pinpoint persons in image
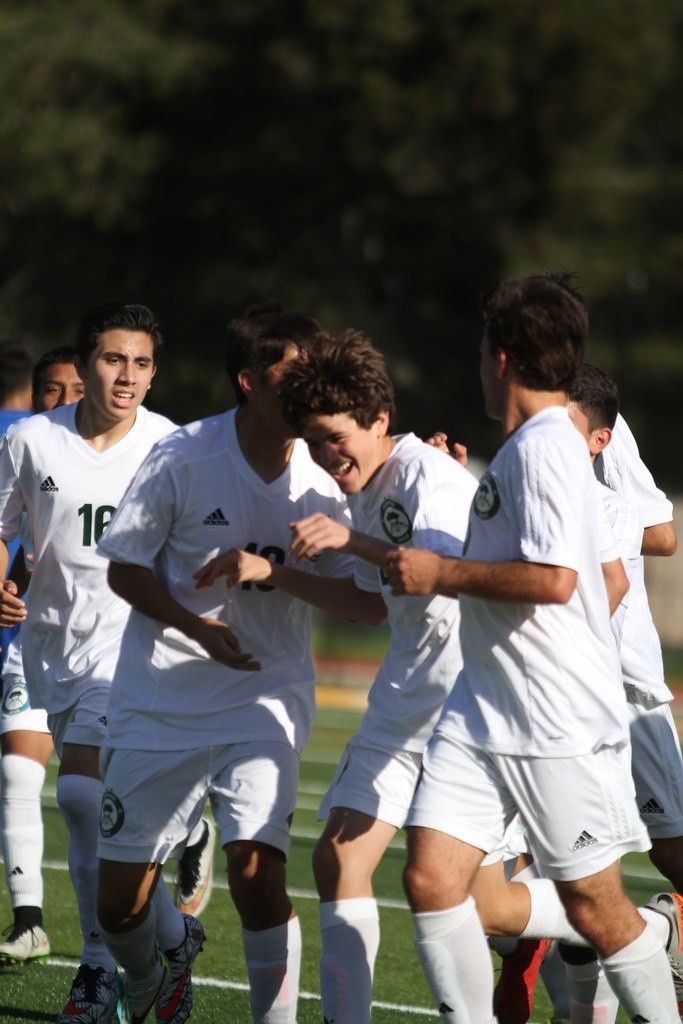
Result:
[0,277,683,1024]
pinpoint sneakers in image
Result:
[153,915,208,1024]
[57,964,122,1024]
[172,818,216,918]
[493,939,553,1024]
[0,923,50,966]
[645,892,683,1021]
[122,950,170,1024]
